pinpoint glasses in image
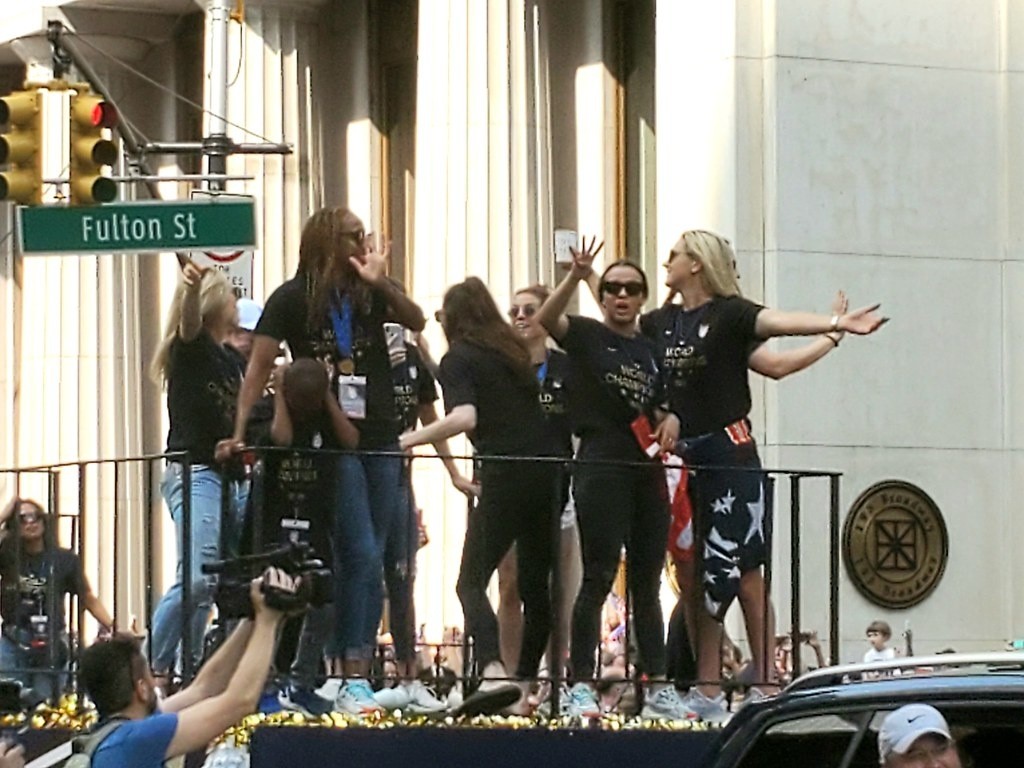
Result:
[435,310,446,322]
[507,307,535,318]
[230,287,241,299]
[668,250,694,263]
[603,281,644,298]
[336,229,365,246]
[19,511,44,524]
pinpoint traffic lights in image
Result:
[0,92,44,207]
[69,95,119,207]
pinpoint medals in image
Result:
[337,358,354,374]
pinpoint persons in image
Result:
[879,702,964,768]
[1,206,890,716]
[862,621,1018,679]
[72,564,298,767]
[0,741,25,768]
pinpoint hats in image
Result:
[236,298,263,331]
[878,704,951,764]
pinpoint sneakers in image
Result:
[254,672,769,727]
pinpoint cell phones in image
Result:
[383,324,404,355]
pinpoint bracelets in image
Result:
[826,334,838,346]
[831,315,838,329]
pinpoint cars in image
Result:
[692,650,1024,768]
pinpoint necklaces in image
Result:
[678,298,709,346]
[666,307,709,378]
